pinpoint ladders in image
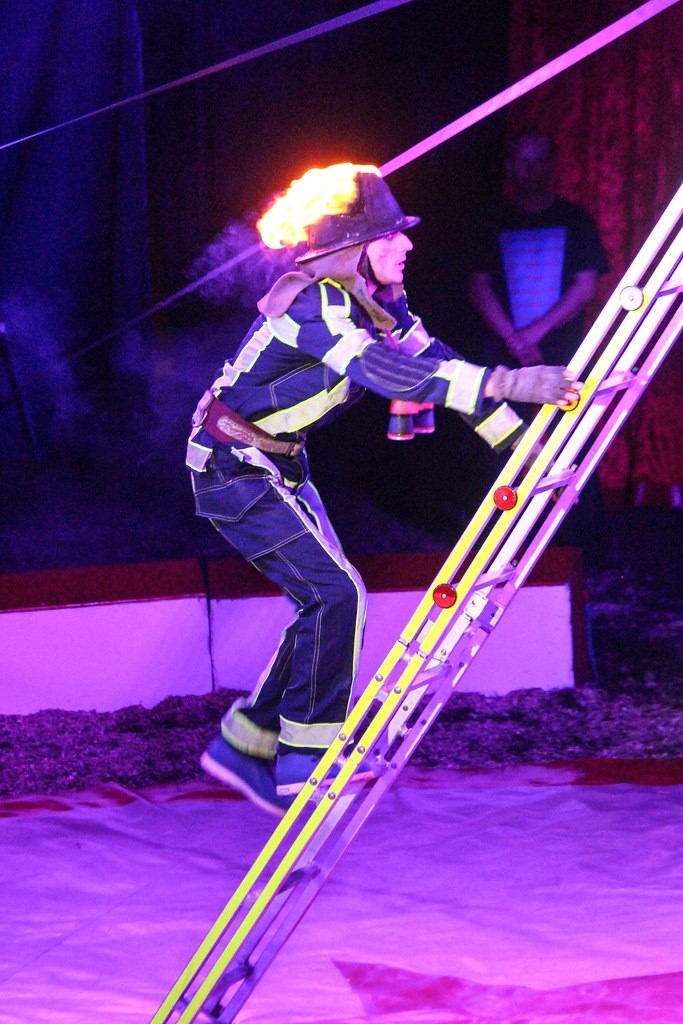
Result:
[147,188,683,1024]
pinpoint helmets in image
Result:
[295,172,421,263]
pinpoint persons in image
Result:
[184,161,594,818]
[460,127,611,557]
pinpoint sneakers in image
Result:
[201,735,295,817]
[275,742,374,797]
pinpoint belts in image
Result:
[216,416,303,457]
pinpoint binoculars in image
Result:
[386,398,435,440]
